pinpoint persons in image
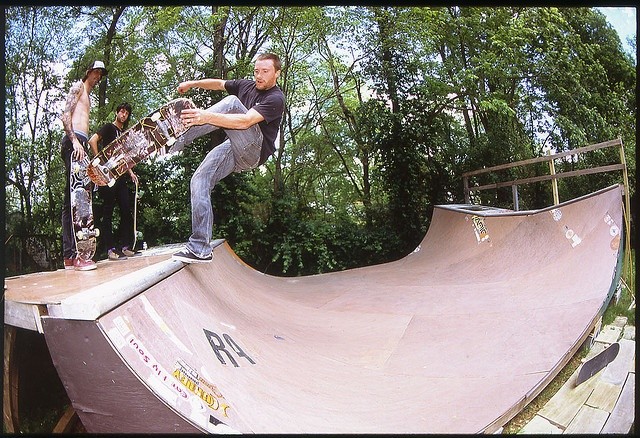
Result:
[156,52,287,264]
[61,60,109,271]
[87,103,143,260]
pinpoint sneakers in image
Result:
[64,257,74,269]
[73,258,97,270]
[122,246,142,256]
[159,145,170,155]
[108,247,127,259]
[172,247,213,263]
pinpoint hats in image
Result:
[88,60,108,75]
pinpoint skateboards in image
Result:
[87,97,199,186]
[70,150,100,262]
[575,343,620,387]
[132,179,144,250]
